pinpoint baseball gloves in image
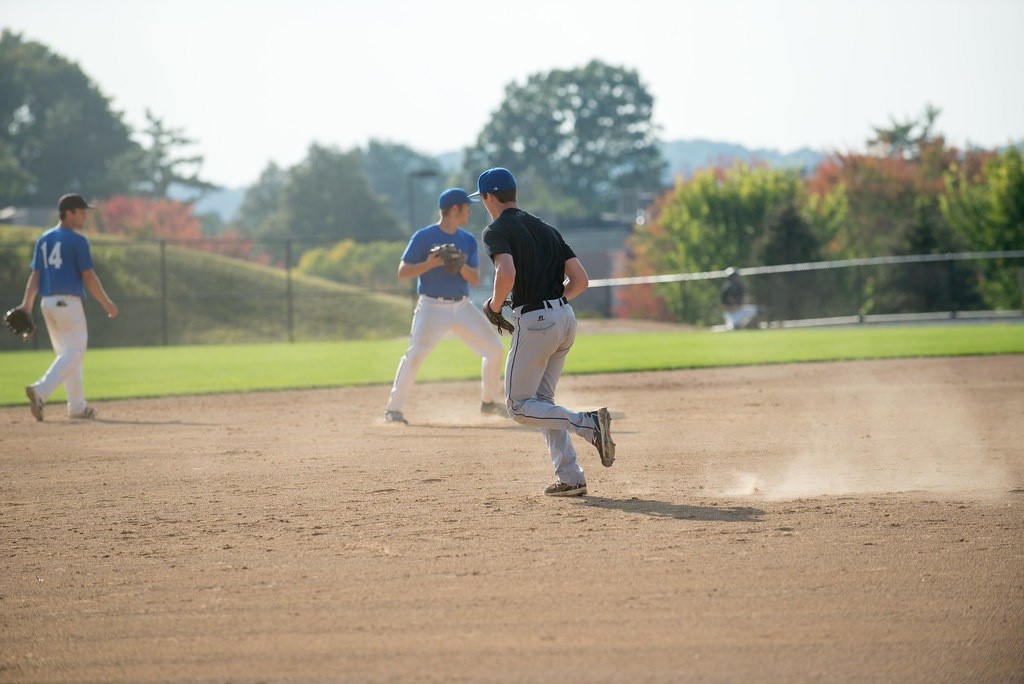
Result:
[3,307,37,341]
[430,244,465,274]
[482,296,516,335]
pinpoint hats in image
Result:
[439,188,480,207]
[467,168,515,199]
[58,194,95,210]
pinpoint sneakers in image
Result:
[27,386,43,422]
[69,405,98,420]
[585,407,615,467]
[544,482,587,497]
[385,411,408,426]
[480,402,511,419]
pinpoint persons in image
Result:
[467,167,617,495]
[387,189,508,425]
[4,193,119,421]
[713,268,757,333]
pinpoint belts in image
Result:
[520,297,568,315]
[425,294,462,302]
